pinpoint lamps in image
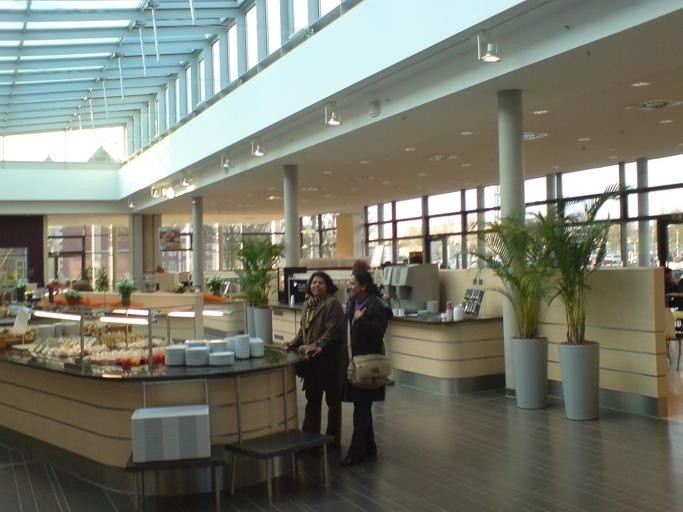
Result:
[220,152,231,169]
[127,197,135,208]
[474,31,500,62]
[250,138,264,157]
[323,104,342,128]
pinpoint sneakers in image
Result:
[342,447,376,466]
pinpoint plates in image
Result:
[408,300,439,318]
[164,335,264,368]
[12,344,29,351]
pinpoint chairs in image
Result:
[225,365,335,508]
[665,307,682,372]
[125,378,226,511]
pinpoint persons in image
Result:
[282,270,348,451]
[663,266,679,308]
[346,258,395,386]
[338,269,391,468]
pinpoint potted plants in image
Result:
[206,276,224,297]
[116,272,136,306]
[528,183,632,421]
[235,237,285,343]
[451,217,553,410]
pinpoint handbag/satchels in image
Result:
[348,354,391,389]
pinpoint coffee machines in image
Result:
[382,263,441,314]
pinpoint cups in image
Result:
[440,303,464,322]
[408,251,424,264]
[391,308,407,318]
[289,295,295,307]
